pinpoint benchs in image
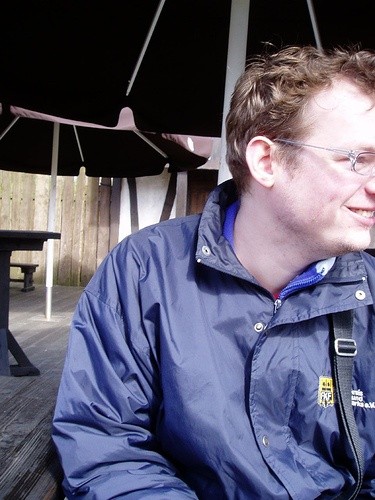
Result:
[9,263,41,293]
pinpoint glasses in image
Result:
[271,137,375,175]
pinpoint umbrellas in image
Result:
[0,0,375,184]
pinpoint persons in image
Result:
[51,45,375,500]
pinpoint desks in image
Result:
[0,229,63,378]
